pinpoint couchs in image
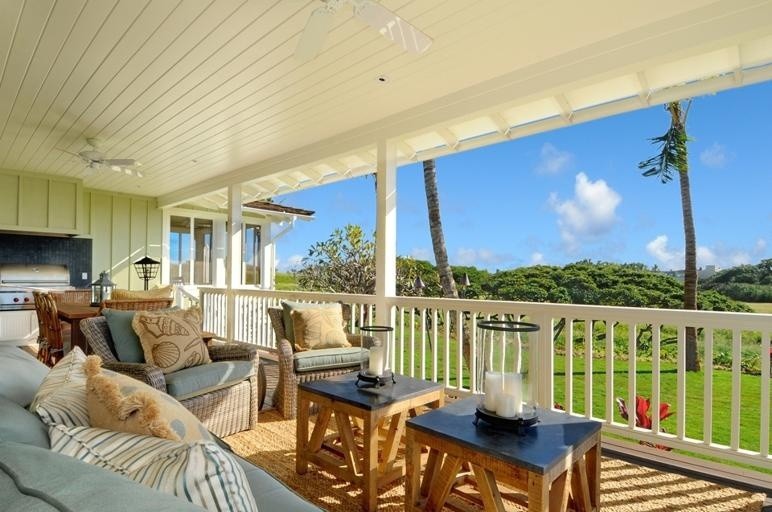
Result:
[0,344,325,512]
[79,309,261,440]
[267,302,381,419]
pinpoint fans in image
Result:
[60,138,146,181]
[292,1,434,60]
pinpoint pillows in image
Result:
[287,307,353,351]
[45,421,257,512]
[102,311,146,363]
[29,344,112,429]
[280,299,341,350]
[133,310,213,374]
[83,355,216,441]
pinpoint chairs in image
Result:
[30,288,45,362]
[103,297,174,312]
[42,292,64,367]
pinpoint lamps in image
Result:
[133,257,161,291]
